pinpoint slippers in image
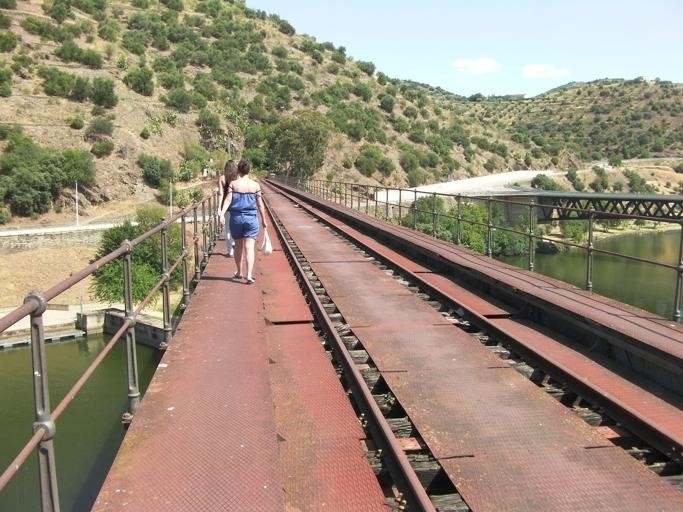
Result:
[234,271,255,284]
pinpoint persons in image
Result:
[219,160,239,259]
[219,159,267,284]
